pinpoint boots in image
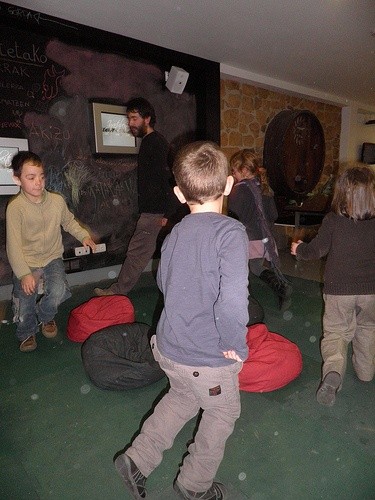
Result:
[258,267,294,312]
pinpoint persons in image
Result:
[226,148,295,324]
[113,140,250,500]
[4,150,97,354]
[290,164,374,409]
[93,96,179,299]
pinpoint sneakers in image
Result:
[114,453,147,500]
[19,334,36,353]
[315,371,341,408]
[41,318,57,338]
[171,480,226,500]
[95,287,123,299]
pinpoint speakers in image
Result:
[164,65,189,94]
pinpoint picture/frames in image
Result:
[87,96,143,160]
[0,137,30,196]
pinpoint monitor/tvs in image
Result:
[91,101,141,157]
